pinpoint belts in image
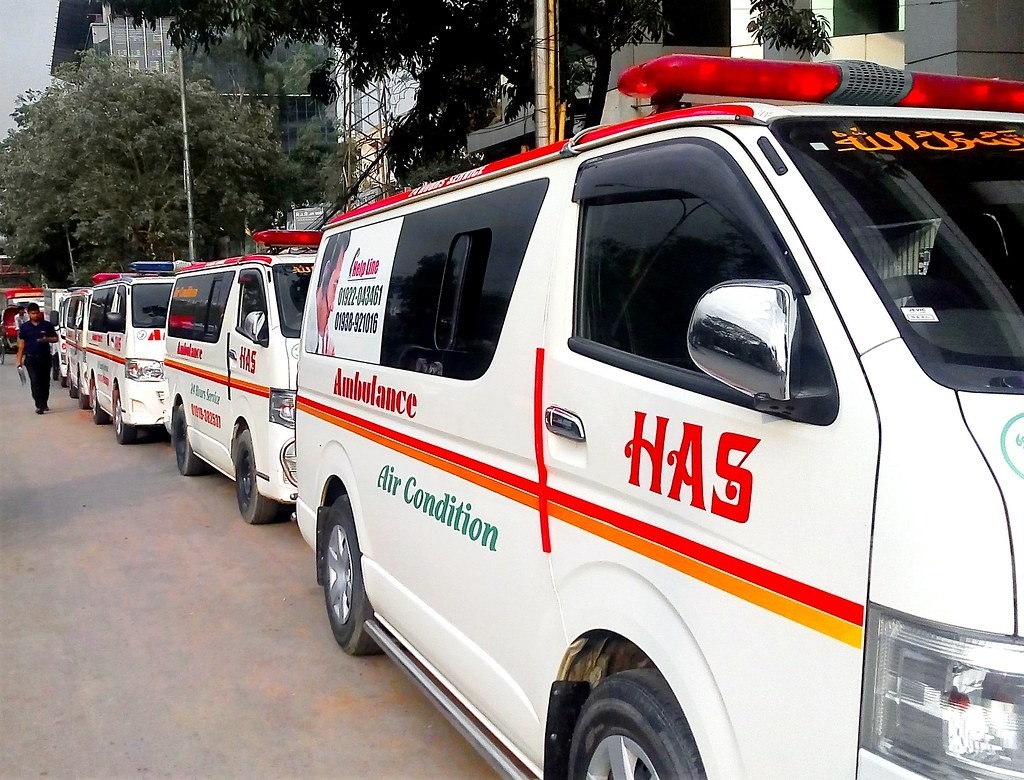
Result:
[26,354,48,357]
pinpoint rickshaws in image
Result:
[0,306,31,364]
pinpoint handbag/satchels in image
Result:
[17,366,26,383]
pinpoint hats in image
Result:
[18,306,25,311]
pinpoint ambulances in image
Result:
[0,260,206,446]
[162,230,327,526]
[293,53,1024,780]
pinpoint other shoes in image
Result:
[35,408,43,414]
[43,407,48,411]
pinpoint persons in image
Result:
[14,306,30,331]
[17,302,59,414]
[306,230,352,355]
